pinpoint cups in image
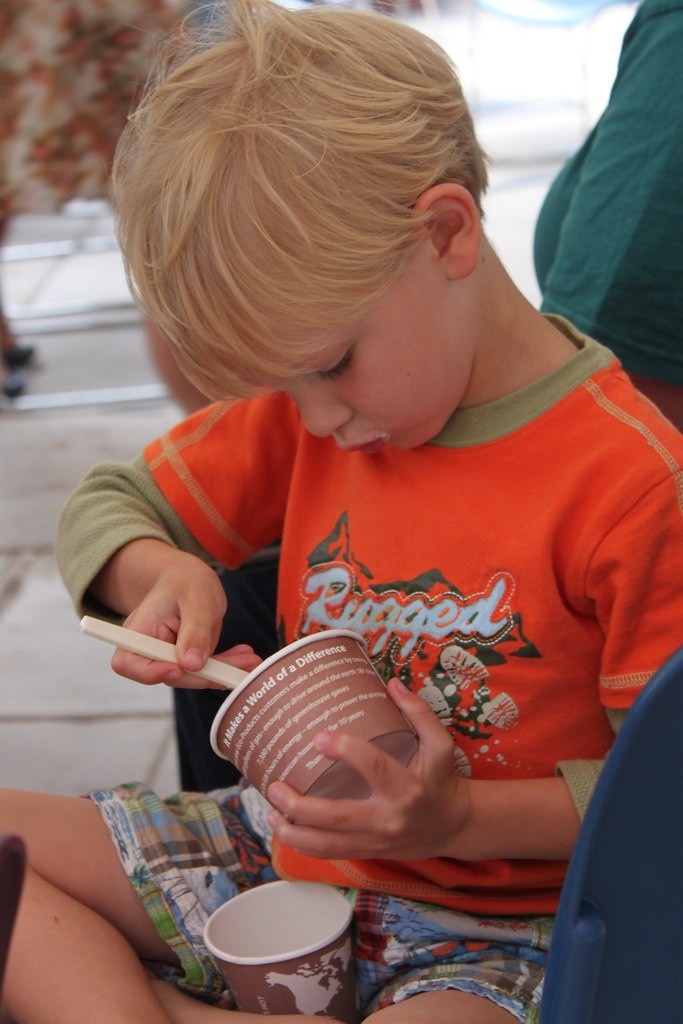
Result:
[202,880,364,1024]
[209,628,419,825]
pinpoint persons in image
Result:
[168,0,682,791]
[0,0,683,1024]
